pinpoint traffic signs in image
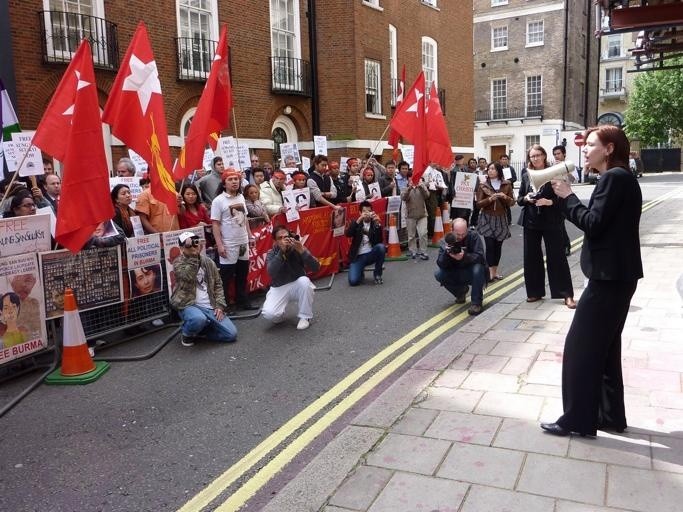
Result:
[574,135,586,147]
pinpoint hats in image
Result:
[220,168,241,184]
[454,154,464,160]
[176,231,199,246]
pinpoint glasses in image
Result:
[15,202,35,207]
[529,153,543,158]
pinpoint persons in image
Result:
[35,174,62,211]
[261,225,320,330]
[200,155,225,211]
[107,160,145,235]
[107,185,157,236]
[177,184,210,227]
[433,217,485,315]
[238,183,272,226]
[521,150,533,171]
[337,158,359,201]
[365,153,386,182]
[132,267,156,297]
[377,161,400,196]
[496,155,517,223]
[402,172,429,261]
[539,125,643,439]
[551,148,581,257]
[5,193,35,218]
[38,154,52,180]
[289,171,309,190]
[395,160,409,196]
[327,160,346,202]
[423,162,443,239]
[171,231,239,348]
[283,156,299,172]
[443,155,486,226]
[474,163,513,283]
[135,183,182,232]
[309,156,343,212]
[333,208,345,230]
[343,201,387,286]
[358,167,375,200]
[0,180,26,206]
[260,170,292,214]
[0,272,40,350]
[243,156,266,188]
[210,168,259,315]
[519,146,579,311]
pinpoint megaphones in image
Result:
[525,161,576,194]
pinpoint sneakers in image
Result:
[469,225,475,230]
[271,318,281,324]
[87,346,95,359]
[468,302,483,315]
[361,271,365,280]
[411,252,416,259]
[297,319,310,329]
[95,339,106,346]
[419,253,429,260]
[374,275,384,284]
[158,319,164,325]
[454,284,468,303]
[181,333,194,347]
[153,320,160,326]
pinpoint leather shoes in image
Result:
[564,297,575,309]
[237,303,260,310]
[526,296,541,301]
[135,326,145,332]
[541,422,592,438]
[487,273,495,282]
[123,327,136,336]
[596,423,625,433]
[495,272,503,279]
[225,306,235,316]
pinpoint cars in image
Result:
[596,154,644,182]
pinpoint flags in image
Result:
[32,39,114,255]
[170,22,231,182]
[102,19,179,215]
[391,69,426,185]
[413,80,452,185]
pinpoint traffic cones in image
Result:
[427,207,445,248]
[382,212,409,262]
[442,202,452,235]
[43,288,113,385]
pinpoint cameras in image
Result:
[188,239,205,246]
[445,232,462,254]
[366,211,374,218]
[288,231,300,240]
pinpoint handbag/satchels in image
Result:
[484,178,512,225]
